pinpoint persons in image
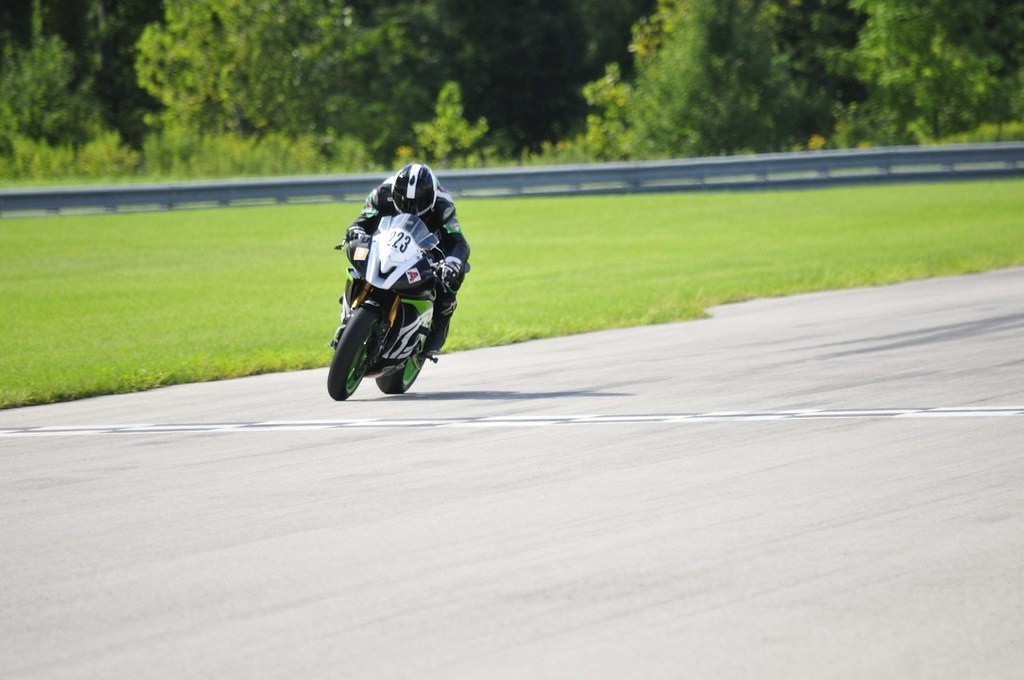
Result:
[345,163,471,354]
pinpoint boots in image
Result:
[424,302,456,352]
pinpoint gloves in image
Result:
[344,225,368,245]
[434,256,463,285]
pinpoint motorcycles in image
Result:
[327,213,470,403]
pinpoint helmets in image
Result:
[389,163,438,216]
